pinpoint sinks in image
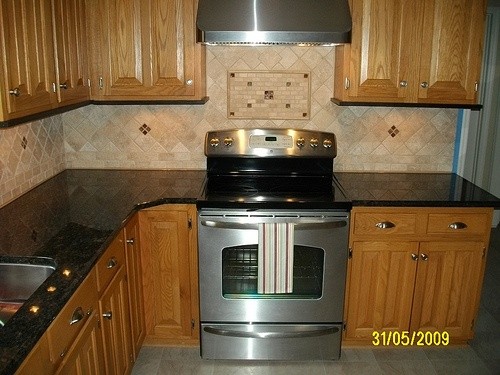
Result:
[0,255,57,329]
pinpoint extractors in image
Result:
[195,0,353,46]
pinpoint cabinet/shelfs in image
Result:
[345,208,489,348]
[86,2,196,96]
[334,0,488,103]
[140,208,190,337]
[0,0,86,114]
[19,215,144,372]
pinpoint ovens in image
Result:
[197,214,351,360]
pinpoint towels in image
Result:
[257,223,294,294]
